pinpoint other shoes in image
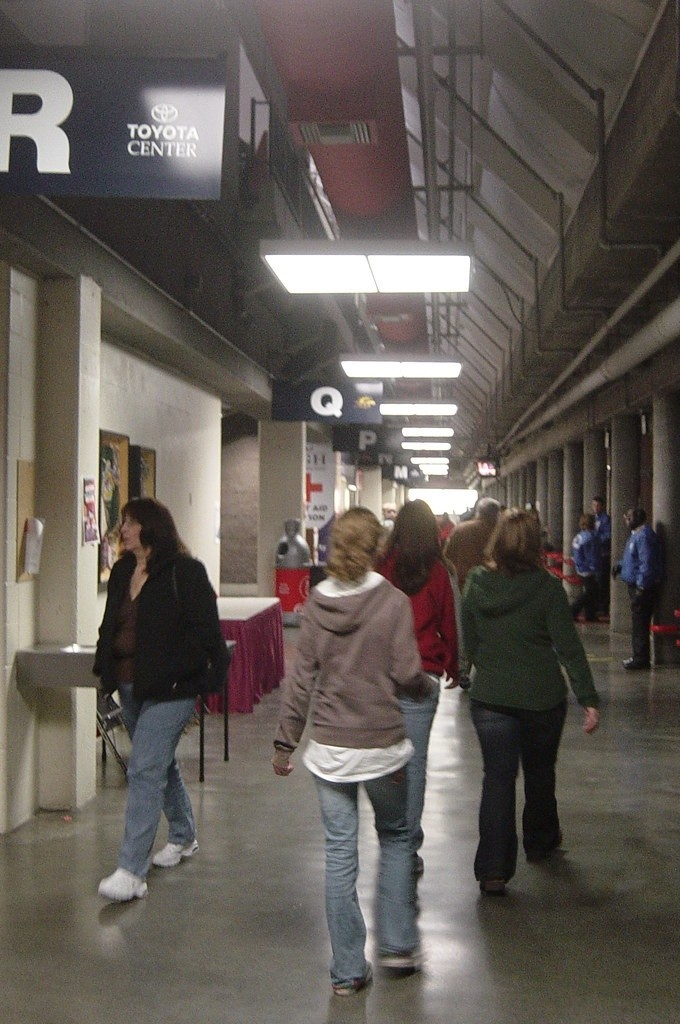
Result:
[527,830,562,858]
[479,880,504,892]
[623,657,650,669]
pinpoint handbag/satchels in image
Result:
[172,556,230,693]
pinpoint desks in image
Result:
[196,596,286,715]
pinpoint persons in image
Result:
[375,495,459,873]
[274,519,311,568]
[610,506,662,672]
[455,507,603,895]
[273,506,434,995]
[435,498,508,594]
[92,499,229,902]
[568,496,610,623]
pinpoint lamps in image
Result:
[337,352,463,379]
[259,237,471,294]
[380,398,459,417]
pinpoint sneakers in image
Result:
[98,867,148,901]
[152,838,199,866]
[376,949,422,968]
[335,962,372,996]
